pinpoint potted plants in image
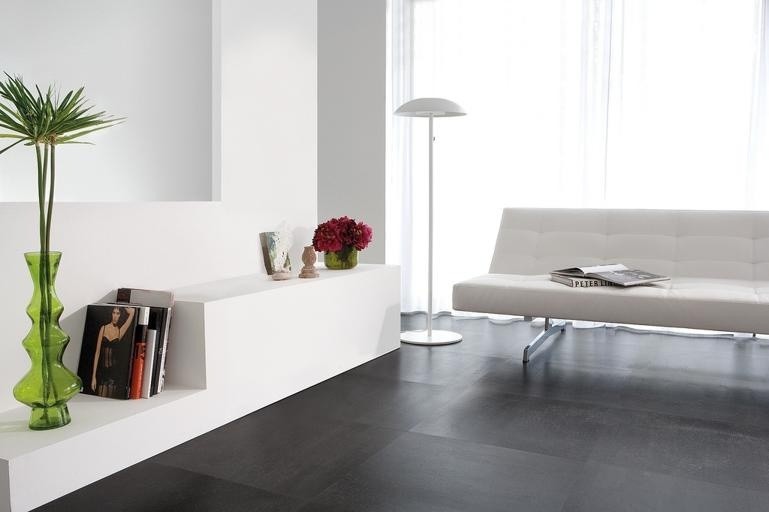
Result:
[0,72,128,434]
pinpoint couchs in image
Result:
[453,207,769,367]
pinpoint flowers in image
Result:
[312,213,374,270]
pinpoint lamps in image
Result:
[392,96,466,346]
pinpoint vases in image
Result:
[322,245,359,269]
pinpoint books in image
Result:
[72,288,179,403]
[259,230,294,275]
[550,262,673,287]
[550,275,617,289]
